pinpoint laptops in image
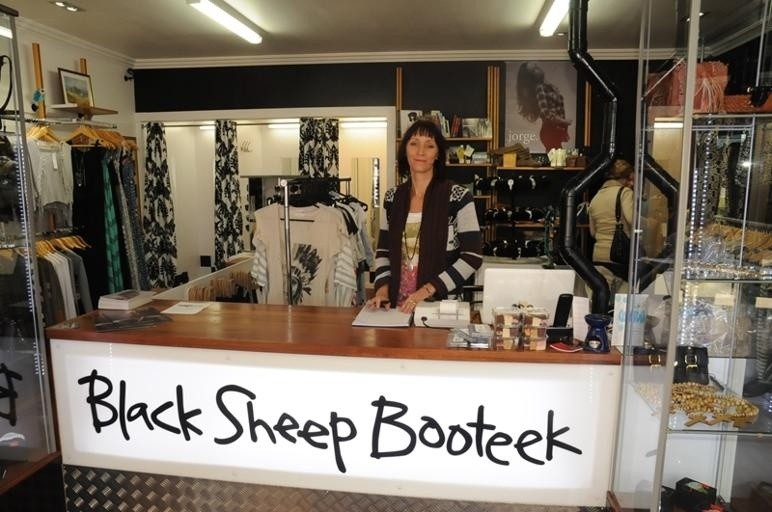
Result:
[479,268,577,325]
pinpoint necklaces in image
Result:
[402,220,421,271]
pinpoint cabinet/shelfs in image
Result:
[395,133,586,261]
[603,109,772,512]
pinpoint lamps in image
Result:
[187,0,265,45]
[538,0,570,38]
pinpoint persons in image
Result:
[588,160,634,282]
[366,121,484,314]
[517,62,573,152]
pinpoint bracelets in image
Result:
[423,284,432,298]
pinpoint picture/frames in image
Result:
[57,67,95,107]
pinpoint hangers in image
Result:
[257,174,368,217]
[0,229,94,265]
[188,269,256,302]
[20,120,71,149]
[63,120,140,151]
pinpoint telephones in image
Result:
[414,300,471,328]
